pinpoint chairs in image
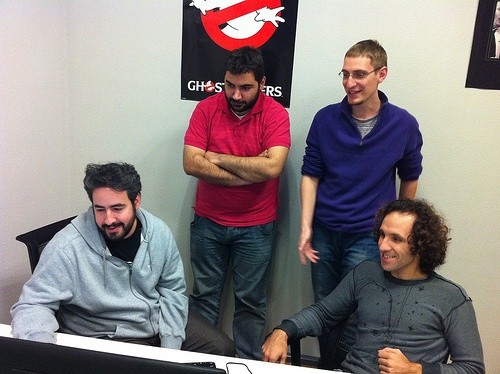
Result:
[16,216,77,275]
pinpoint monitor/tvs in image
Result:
[0,337,227,374]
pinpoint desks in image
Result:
[0,324,350,374]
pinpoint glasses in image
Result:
[338,65,385,80]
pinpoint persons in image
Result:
[183,46,292,362]
[261,197,486,374]
[10,161,237,357]
[298,39,423,369]
